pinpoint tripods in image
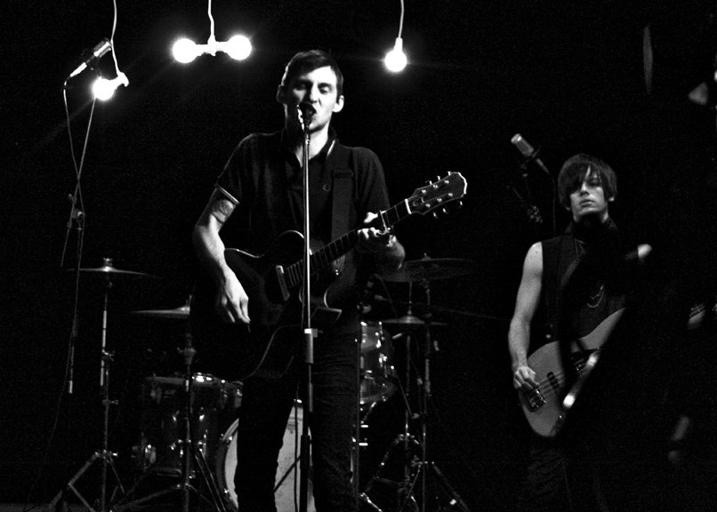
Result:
[108,324,227,512]
[360,284,465,512]
[48,279,126,512]
[399,292,471,512]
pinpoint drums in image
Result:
[141,372,235,477]
[216,399,361,507]
[359,321,397,405]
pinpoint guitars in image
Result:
[188,171,467,387]
[517,303,716,438]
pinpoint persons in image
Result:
[190,50,406,511]
[506,154,686,512]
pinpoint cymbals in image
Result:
[379,258,478,282]
[127,306,190,321]
[377,316,447,326]
[73,267,153,277]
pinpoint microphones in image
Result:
[510,133,549,173]
[67,38,113,80]
[296,101,316,128]
